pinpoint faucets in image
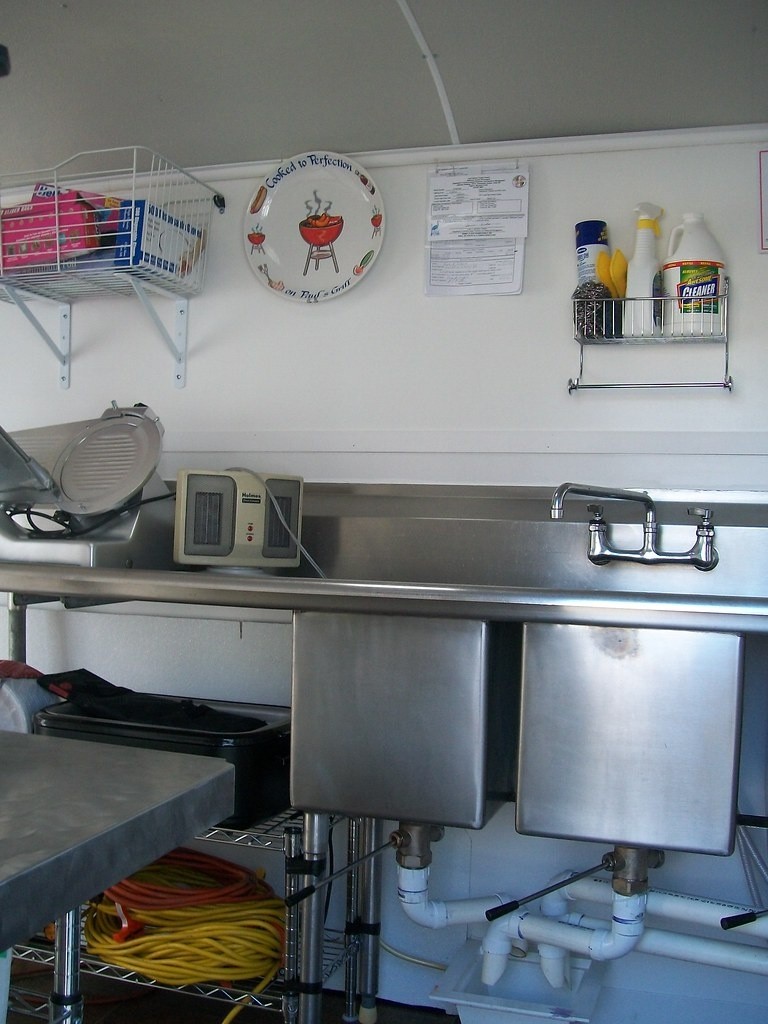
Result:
[551,483,658,553]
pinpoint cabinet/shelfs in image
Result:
[9,810,360,1024]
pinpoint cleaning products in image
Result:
[662,210,729,338]
[620,202,665,338]
[576,218,613,340]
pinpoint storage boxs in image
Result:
[29,696,296,828]
[0,141,220,303]
[567,276,729,342]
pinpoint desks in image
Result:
[0,730,242,1024]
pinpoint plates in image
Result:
[243,150,385,303]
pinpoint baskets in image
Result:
[0,146,223,306]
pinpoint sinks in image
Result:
[289,573,511,831]
[516,585,745,857]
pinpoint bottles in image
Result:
[624,202,665,336]
[662,212,725,335]
